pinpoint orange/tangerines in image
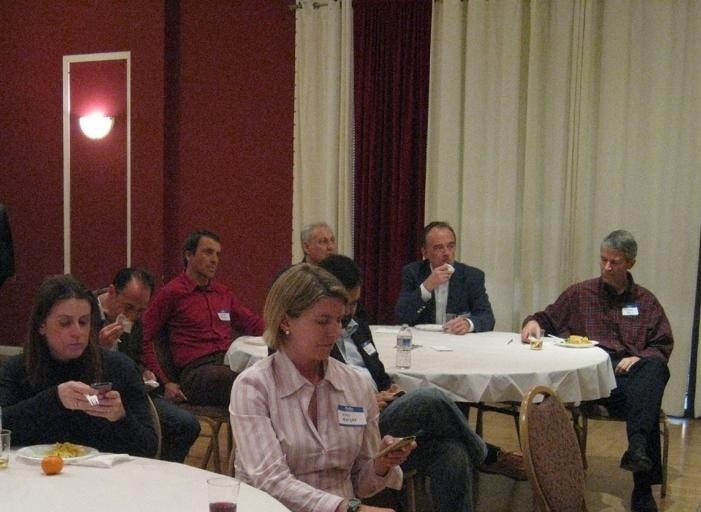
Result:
[40,456,62,476]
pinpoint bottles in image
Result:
[396,324,413,370]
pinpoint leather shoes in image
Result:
[618,450,654,472]
[480,441,529,481]
[630,489,658,512]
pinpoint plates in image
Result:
[415,324,444,331]
[246,337,265,345]
[557,340,599,348]
[16,444,98,465]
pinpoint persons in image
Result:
[521,229,674,512]
[393,222,495,418]
[322,257,527,512]
[87,269,201,463]
[143,228,266,408]
[228,263,417,512]
[0,275,159,457]
[278,223,337,266]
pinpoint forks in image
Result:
[83,393,99,406]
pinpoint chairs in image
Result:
[519,384,589,512]
[573,401,669,498]
[154,334,230,473]
[147,395,162,460]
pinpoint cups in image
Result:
[207,477,240,512]
[0,430,10,468]
[91,382,113,395]
[443,313,455,328]
[530,330,545,350]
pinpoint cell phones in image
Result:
[89,380,109,394]
[367,433,419,463]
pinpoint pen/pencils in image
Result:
[507,339,513,345]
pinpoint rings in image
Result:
[73,403,79,409]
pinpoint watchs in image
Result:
[347,497,361,512]
